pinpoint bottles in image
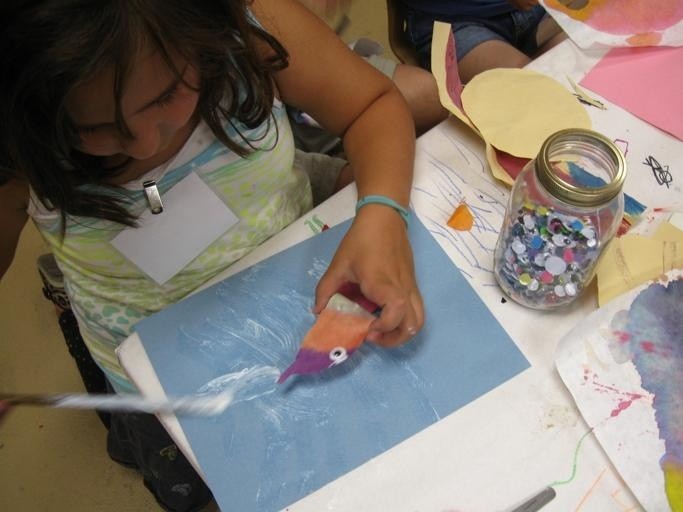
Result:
[491,126,626,311]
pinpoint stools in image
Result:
[34,253,113,436]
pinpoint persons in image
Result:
[284,35,451,208]
[389,0,566,84]
[1,0,426,512]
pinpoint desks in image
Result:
[113,26,683,512]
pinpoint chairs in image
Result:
[383,0,425,71]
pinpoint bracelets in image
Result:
[355,194,412,231]
